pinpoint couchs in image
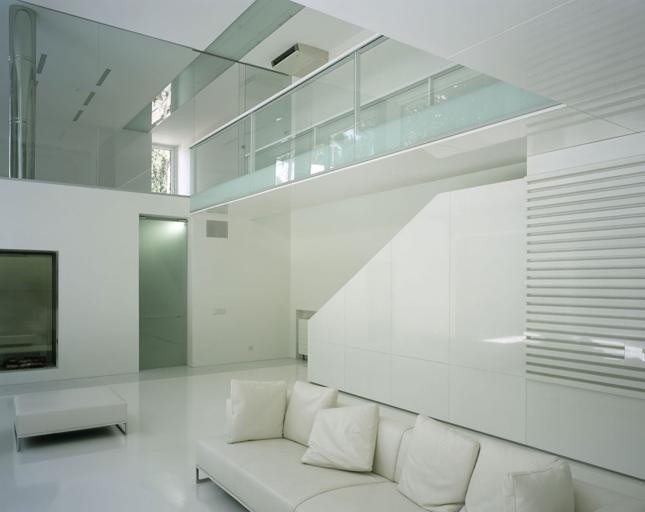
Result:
[196,378,645,512]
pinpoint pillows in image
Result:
[503,457,576,512]
[224,379,378,472]
[396,411,480,512]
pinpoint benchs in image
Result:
[11,385,129,451]
